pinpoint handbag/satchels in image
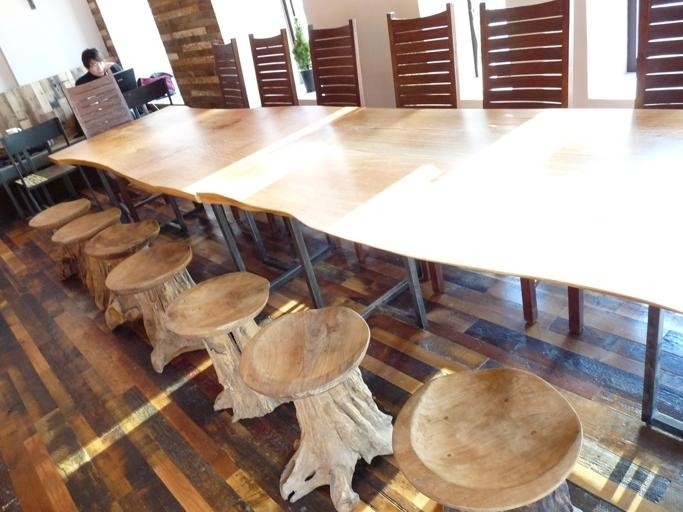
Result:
[137,72,175,99]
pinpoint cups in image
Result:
[4,127,21,137]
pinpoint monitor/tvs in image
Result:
[113,68,137,94]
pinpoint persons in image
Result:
[74,48,145,120]
